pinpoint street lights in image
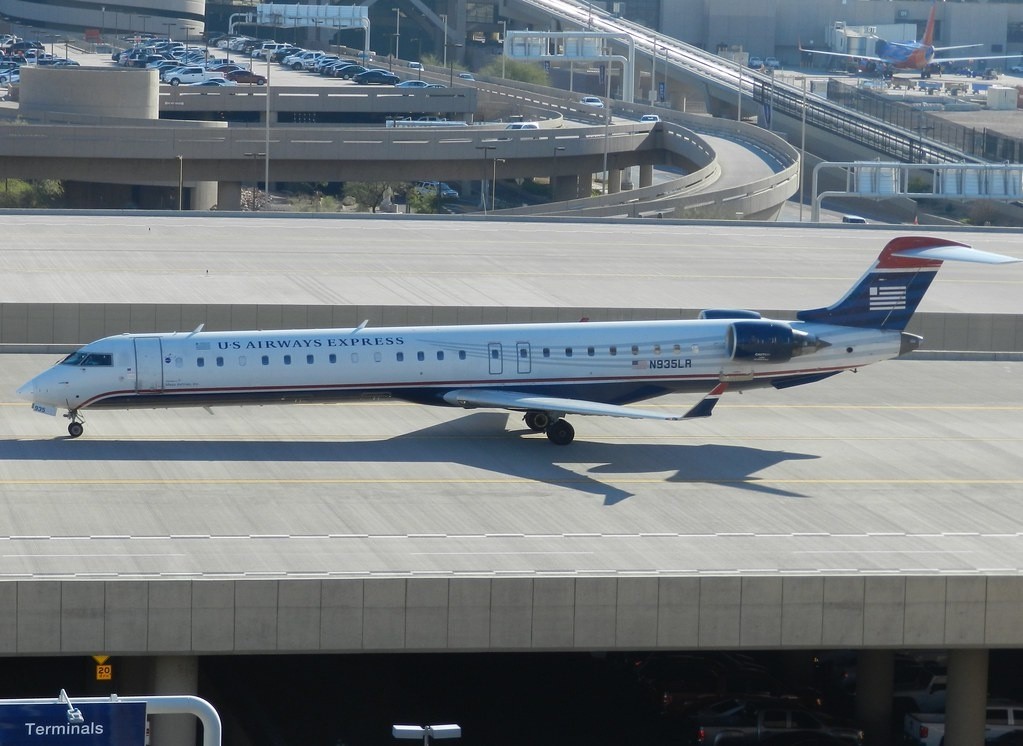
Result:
[176,156,184,211]
[498,21,507,80]
[492,157,505,212]
[440,13,448,68]
[392,7,400,59]
[245,152,266,211]
[731,45,743,122]
[553,146,566,202]
[659,47,668,103]
[794,76,807,222]
[476,145,497,204]
[648,34,657,108]
[600,45,613,194]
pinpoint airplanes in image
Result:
[797,0,1023,80]
[13,226,1023,447]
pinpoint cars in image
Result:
[496,122,540,142]
[408,62,424,73]
[1012,63,1023,73]
[0,16,469,96]
[358,50,373,62]
[981,67,998,81]
[458,72,475,82]
[639,114,662,134]
[579,97,605,110]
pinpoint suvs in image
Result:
[415,179,459,204]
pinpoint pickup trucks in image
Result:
[765,56,780,69]
[749,57,764,68]
